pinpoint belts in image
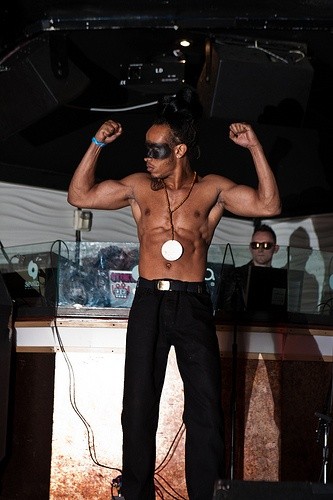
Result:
[139,280,200,293]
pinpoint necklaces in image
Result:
[161,171,197,262]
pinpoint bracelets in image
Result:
[92,137,106,147]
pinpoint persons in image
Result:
[243,224,279,268]
[67,115,282,500]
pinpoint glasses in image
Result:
[250,242,273,250]
